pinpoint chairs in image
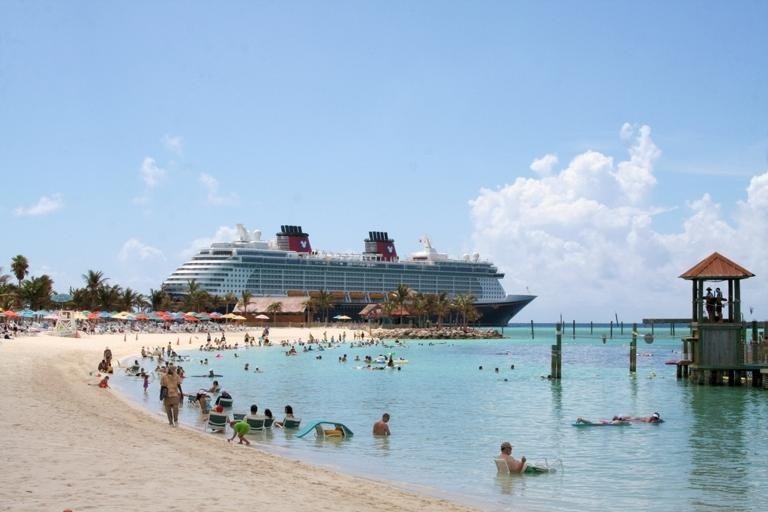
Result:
[206,396,347,441]
[494,459,510,475]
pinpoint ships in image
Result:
[162,224,538,326]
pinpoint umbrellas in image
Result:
[254,313,271,328]
[333,314,352,321]
[0,308,247,321]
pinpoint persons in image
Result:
[3,320,33,337]
[705,287,716,322]
[715,287,724,324]
[196,391,210,414]
[274,405,295,427]
[499,440,557,475]
[477,363,552,381]
[624,412,660,423]
[159,367,185,426]
[762,335,768,363]
[211,405,230,431]
[576,415,625,424]
[372,413,391,436]
[263,408,274,422]
[216,390,233,404]
[122,321,454,392]
[242,404,262,422]
[89,321,95,336]
[98,347,114,388]
[226,419,251,445]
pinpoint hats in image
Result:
[501,441,516,450]
